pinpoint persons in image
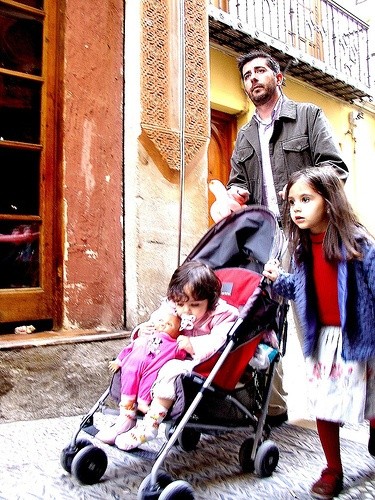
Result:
[107,301,191,433]
[228,49,348,428]
[94,262,242,454]
[262,166,375,496]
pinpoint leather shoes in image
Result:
[253,410,288,433]
[368,433,375,457]
[310,466,344,500]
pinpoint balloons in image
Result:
[209,180,244,222]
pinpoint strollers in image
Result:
[60,205,288,500]
